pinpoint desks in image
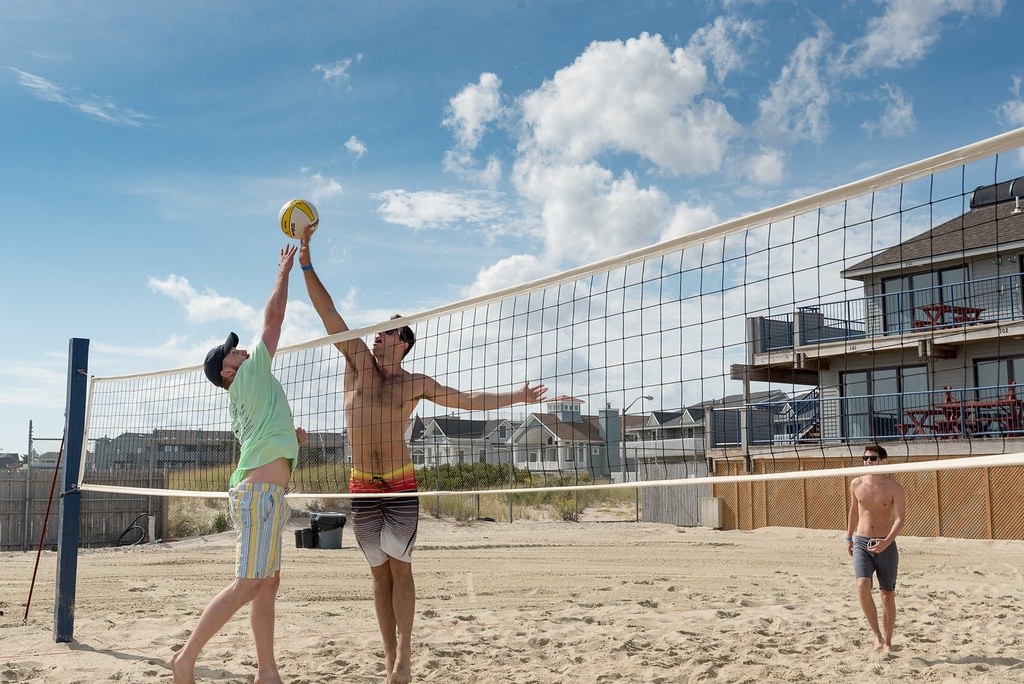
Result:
[906,410,972,441]
[923,305,986,331]
[935,399,1022,439]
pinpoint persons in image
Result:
[846,445,906,654]
[298,222,549,684]
[168,243,302,684]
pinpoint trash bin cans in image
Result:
[294,528,316,548]
[310,512,346,549]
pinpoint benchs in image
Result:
[912,320,946,333]
[953,316,998,327]
[894,417,1023,439]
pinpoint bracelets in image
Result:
[847,537,852,540]
[300,264,313,271]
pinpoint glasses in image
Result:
[861,456,878,461]
[386,328,399,335]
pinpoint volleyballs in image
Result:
[278,198,320,240]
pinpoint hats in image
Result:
[203,332,239,387]
